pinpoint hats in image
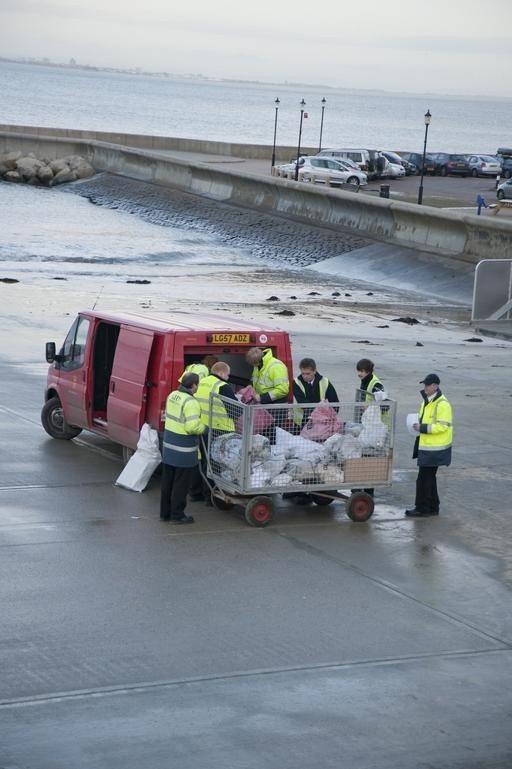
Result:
[357,359,374,370]
[420,374,440,384]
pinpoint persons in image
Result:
[193,361,245,507]
[351,358,390,499]
[405,374,453,517]
[176,354,218,502]
[159,373,206,525]
[281,357,340,499]
[246,347,289,444]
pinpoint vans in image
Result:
[42,309,297,451]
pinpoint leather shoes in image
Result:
[406,509,431,517]
[431,509,438,516]
[191,495,206,501]
[160,516,170,521]
[169,516,192,524]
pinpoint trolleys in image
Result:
[208,394,397,528]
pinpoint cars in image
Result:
[291,135,511,199]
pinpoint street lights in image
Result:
[417,106,432,202]
[318,96,328,153]
[295,98,306,180]
[269,92,280,167]
